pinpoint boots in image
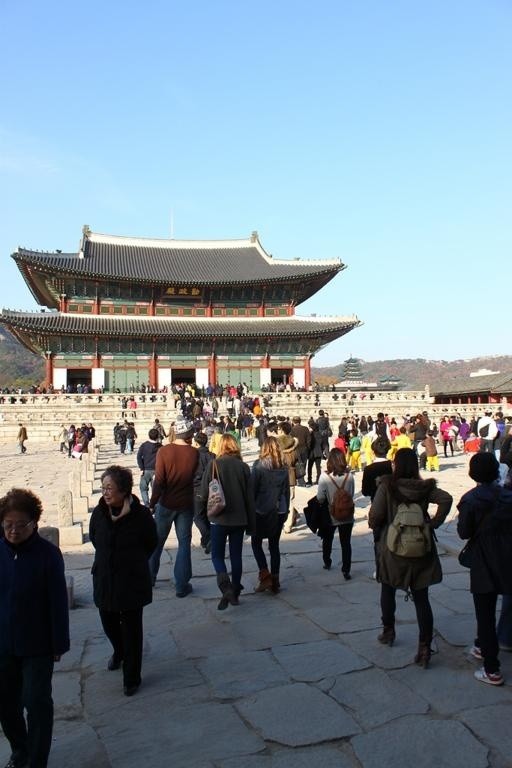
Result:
[217,569,282,610]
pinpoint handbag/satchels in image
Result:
[458,544,485,568]
[207,460,227,518]
[479,424,490,437]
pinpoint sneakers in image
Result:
[468,646,487,666]
[323,563,352,581]
[475,666,504,687]
[497,638,512,652]
[120,449,136,455]
[176,583,195,598]
[19,448,26,455]
[420,453,454,472]
[59,446,82,461]
[307,481,319,486]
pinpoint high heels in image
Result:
[412,642,431,670]
[377,628,397,648]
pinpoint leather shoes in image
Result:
[106,651,122,671]
[123,677,141,697]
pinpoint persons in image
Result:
[56,379,511,612]
[0,488,72,768]
[89,466,159,698]
[16,423,29,454]
[366,446,454,671]
[455,450,512,688]
[495,467,511,657]
[1,383,56,405]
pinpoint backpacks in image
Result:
[383,500,431,558]
[322,469,356,523]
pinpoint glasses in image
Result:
[0,520,35,530]
[100,485,127,495]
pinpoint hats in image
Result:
[173,418,195,439]
[466,451,502,483]
